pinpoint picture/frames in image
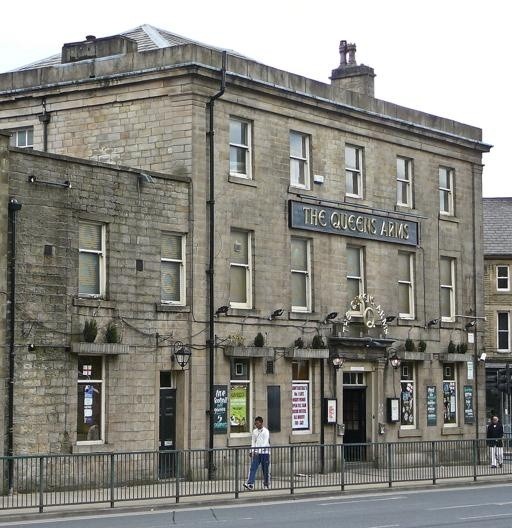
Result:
[322,397,338,425]
[386,397,401,423]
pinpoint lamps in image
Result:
[327,351,346,398]
[387,351,404,397]
[212,306,479,332]
[171,340,192,372]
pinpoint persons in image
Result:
[243,415,270,490]
[486,416,505,468]
